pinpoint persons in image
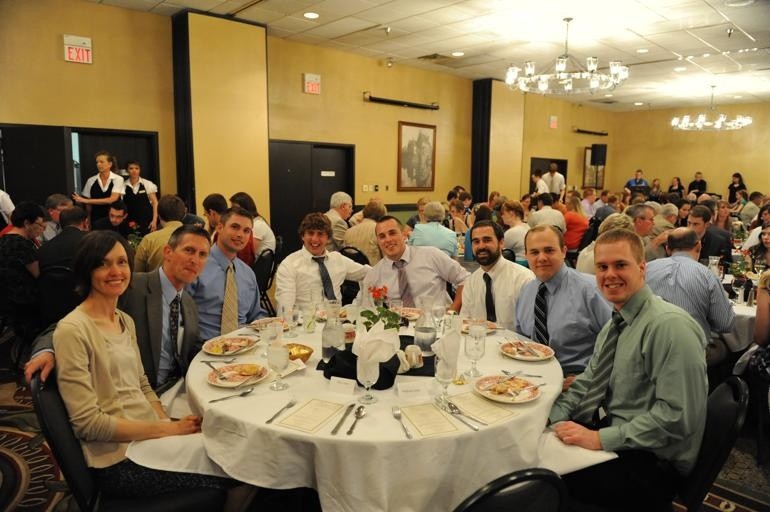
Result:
[543,162,565,203]
[649,179,663,196]
[624,169,649,195]
[667,177,686,199]
[529,168,554,206]
[0,186,769,512]
[69,149,126,230]
[121,159,163,235]
[728,172,747,203]
[688,172,706,196]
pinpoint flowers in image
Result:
[740,247,752,262]
[128,220,138,231]
[730,238,743,249]
[360,285,401,332]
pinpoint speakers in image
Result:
[591,144,607,165]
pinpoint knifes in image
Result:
[443,406,478,431]
[331,405,355,435]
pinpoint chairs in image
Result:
[452,467,566,512]
[339,245,370,305]
[672,374,750,512]
[30,368,226,512]
[250,248,276,317]
[502,249,516,264]
[38,264,75,310]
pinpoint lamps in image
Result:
[505,16,631,100]
[670,82,754,133]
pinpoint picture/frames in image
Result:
[396,119,437,193]
[580,146,605,190]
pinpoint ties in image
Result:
[532,282,550,347]
[549,176,554,193]
[483,272,497,323]
[167,292,184,380]
[571,312,628,425]
[391,259,417,309]
[311,256,338,305]
[220,261,239,337]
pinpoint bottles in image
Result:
[414,298,438,358]
[321,301,346,362]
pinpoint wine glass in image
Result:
[265,341,291,390]
[433,355,457,403]
[708,237,766,307]
[356,356,381,405]
[464,332,486,377]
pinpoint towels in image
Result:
[429,324,462,379]
[351,316,402,380]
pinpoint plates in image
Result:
[201,330,262,356]
[455,317,498,335]
[475,375,541,405]
[315,307,348,323]
[202,357,270,390]
[385,307,424,321]
[498,339,554,362]
[248,318,300,335]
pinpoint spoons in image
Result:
[345,405,366,435]
[266,400,295,422]
[207,387,255,403]
[448,402,488,426]
[393,406,413,439]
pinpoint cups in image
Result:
[389,298,403,327]
[302,302,315,334]
[405,344,424,369]
[455,232,465,255]
